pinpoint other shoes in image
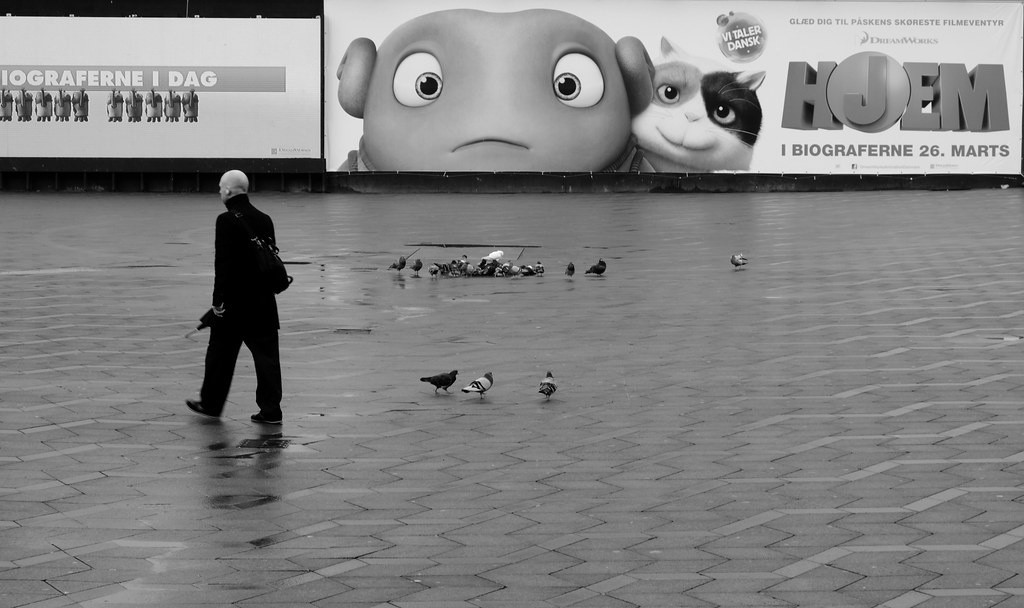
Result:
[186,399,219,419]
[250,409,282,424]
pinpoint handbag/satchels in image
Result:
[266,253,290,295]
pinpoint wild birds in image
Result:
[429,251,544,281]
[388,256,407,273]
[419,369,458,395]
[460,371,494,399]
[539,371,557,400]
[410,258,423,276]
[730,253,747,269]
[585,258,606,277]
[565,262,575,281]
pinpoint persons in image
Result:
[184,170,282,424]
